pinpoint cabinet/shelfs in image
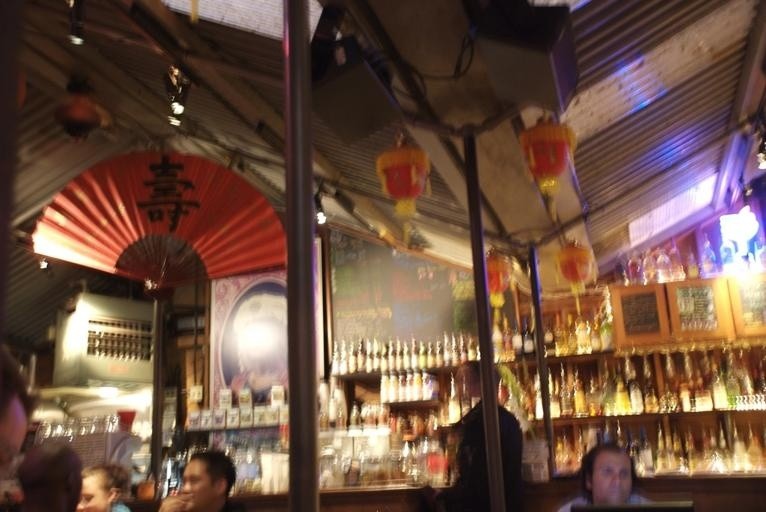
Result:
[325,335,766,489]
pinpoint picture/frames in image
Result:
[664,277,734,344]
[609,283,671,350]
[726,269,765,337]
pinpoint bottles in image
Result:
[330,331,480,376]
[318,402,462,490]
[447,374,482,424]
[490,307,615,362]
[379,368,440,405]
[496,344,764,424]
[555,417,763,478]
[612,229,736,288]
[155,433,289,499]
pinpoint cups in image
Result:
[35,409,137,438]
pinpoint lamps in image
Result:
[160,63,192,129]
[64,0,90,46]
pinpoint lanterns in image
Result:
[556,243,594,297]
[375,145,433,219]
[485,249,512,309]
[520,115,577,198]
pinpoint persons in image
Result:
[444,428,463,478]
[75,462,134,511]
[556,442,652,512]
[18,435,82,509]
[0,344,29,509]
[433,359,524,511]
[157,450,246,511]
